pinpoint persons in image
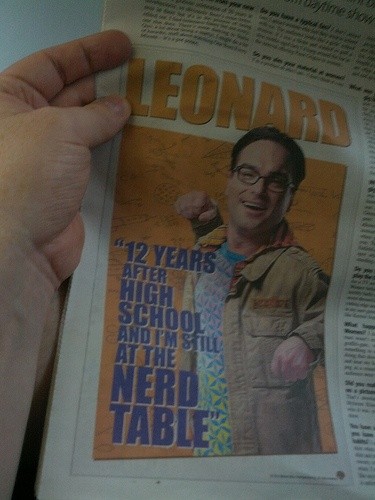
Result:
[173,126,332,457]
[0,30,134,500]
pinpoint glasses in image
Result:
[230,166,295,195]
